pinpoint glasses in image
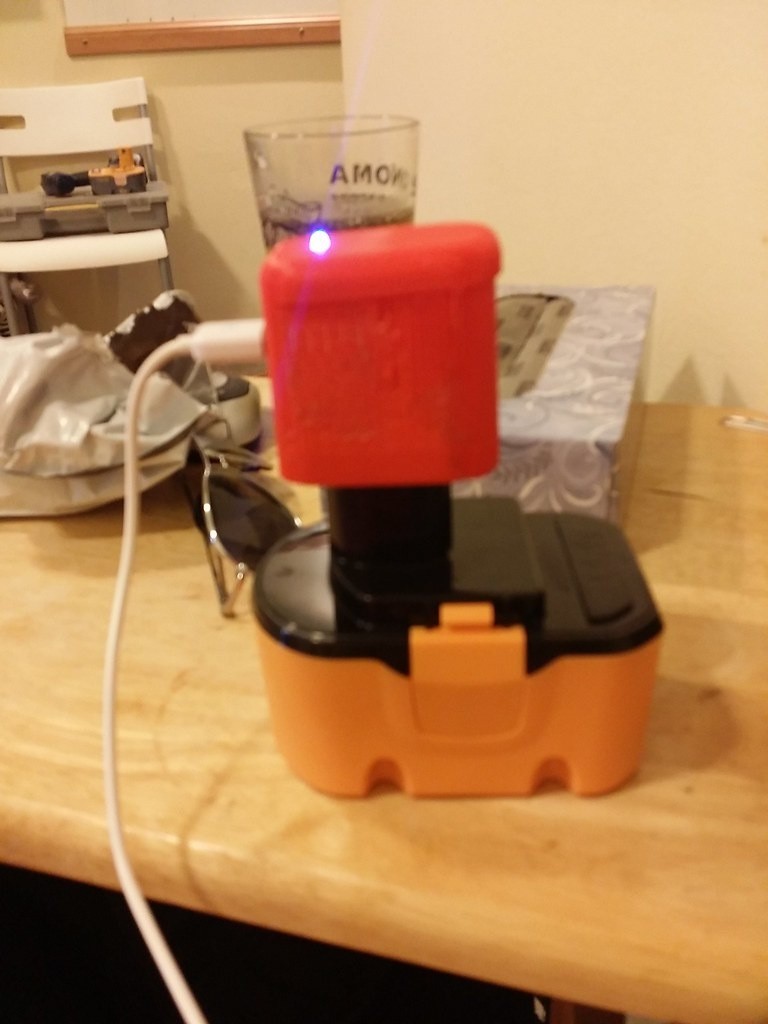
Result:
[188,430,298,619]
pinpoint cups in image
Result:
[242,113,422,253]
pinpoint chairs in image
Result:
[0,77,174,339]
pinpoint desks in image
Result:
[0,371,767,1024]
